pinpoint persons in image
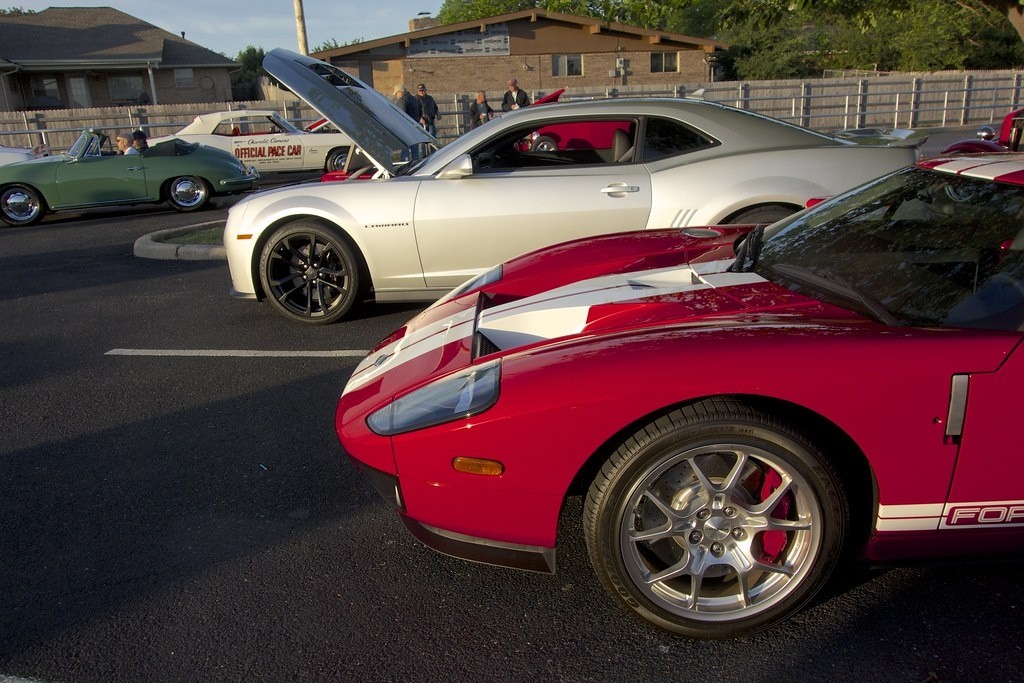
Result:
[115,129,150,155]
[501,78,531,112]
[469,90,494,130]
[391,83,441,162]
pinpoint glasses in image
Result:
[117,140,124,142]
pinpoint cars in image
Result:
[0,126,258,227]
[0,144,50,169]
[942,105,1024,204]
[147,109,353,173]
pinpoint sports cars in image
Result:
[321,88,632,171]
[222,47,928,325]
[333,151,1024,635]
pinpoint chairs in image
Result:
[269,125,276,133]
[610,128,632,162]
[233,126,240,136]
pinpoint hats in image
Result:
[393,84,405,95]
[418,84,425,90]
[132,130,147,139]
[507,79,517,86]
